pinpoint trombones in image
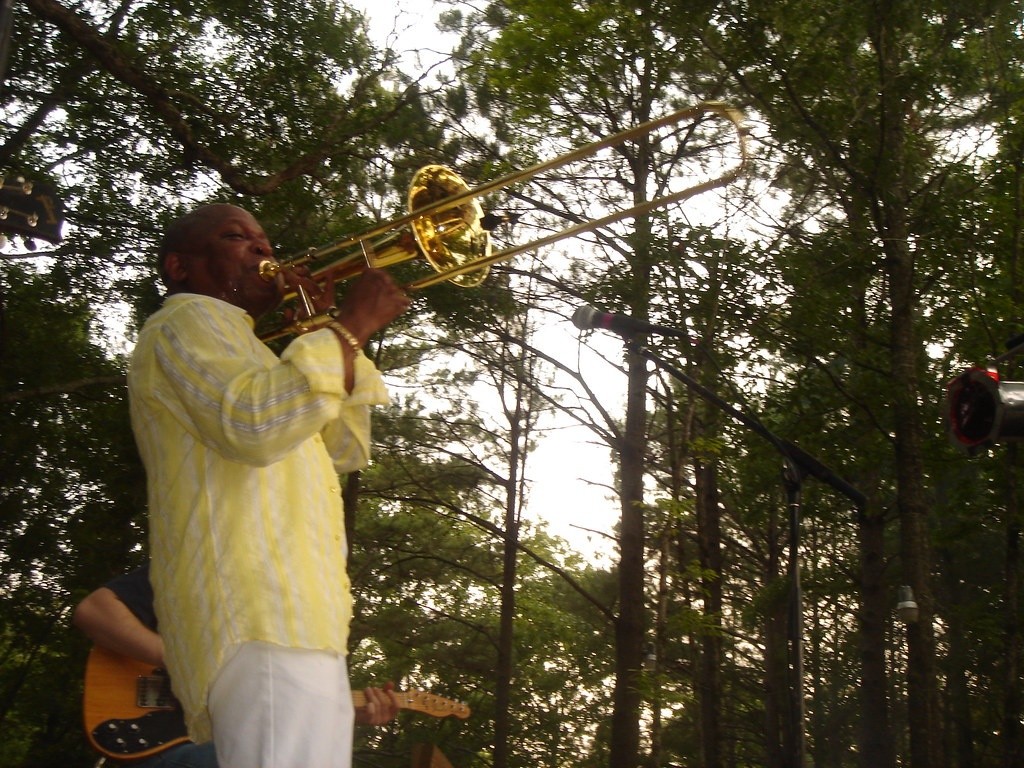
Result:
[249,99,752,344]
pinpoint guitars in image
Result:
[81,634,474,762]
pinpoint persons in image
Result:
[128,202,413,768]
[73,520,398,768]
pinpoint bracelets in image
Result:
[328,320,364,356]
